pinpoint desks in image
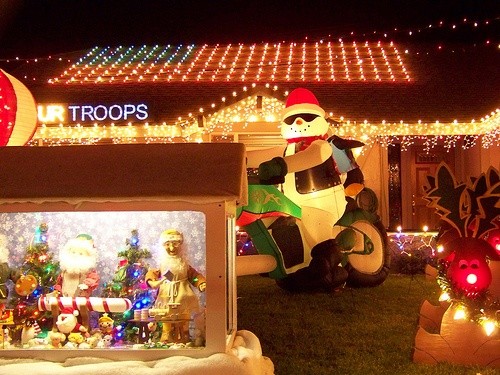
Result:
[127,317,193,345]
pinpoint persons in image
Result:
[144,228,208,348]
[53,232,100,335]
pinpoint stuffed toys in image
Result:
[2,315,120,349]
[57,306,91,344]
